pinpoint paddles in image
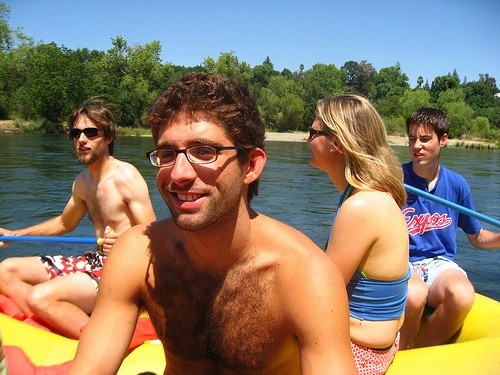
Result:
[0,235,105,245]
[405,185,500,226]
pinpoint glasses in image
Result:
[70,128,104,139]
[145,145,244,168]
[307,127,329,138]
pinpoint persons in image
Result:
[307,94,412,375]
[399,107,500,354]
[0,96,157,339]
[67,73,358,375]
